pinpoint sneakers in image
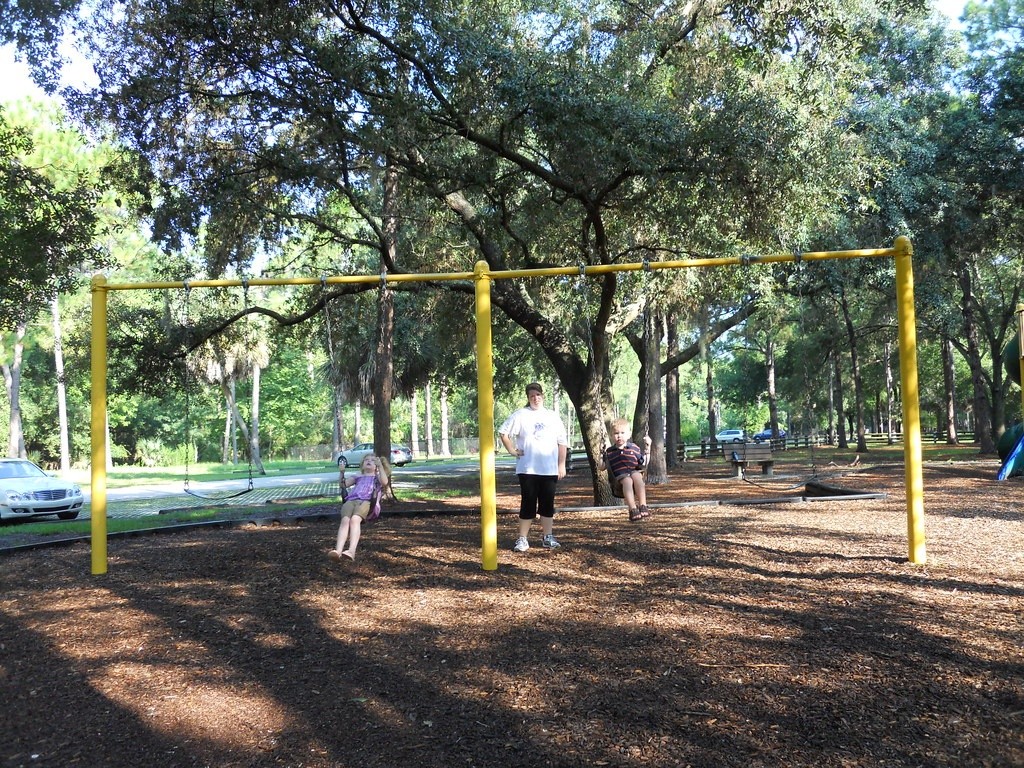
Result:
[542,535,561,549]
[514,536,529,552]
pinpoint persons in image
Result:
[327,452,392,563]
[597,418,652,522]
[498,382,570,552]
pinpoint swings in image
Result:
[319,275,388,522]
[741,252,819,492]
[182,279,254,502]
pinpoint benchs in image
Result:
[722,443,777,478]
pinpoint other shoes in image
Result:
[342,550,356,562]
[330,551,342,565]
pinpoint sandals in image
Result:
[628,509,642,521]
[639,505,650,517]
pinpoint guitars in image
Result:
[576,262,652,501]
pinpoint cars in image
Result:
[332,442,413,467]
[706,430,751,444]
[0,458,84,520]
[753,427,787,443]
